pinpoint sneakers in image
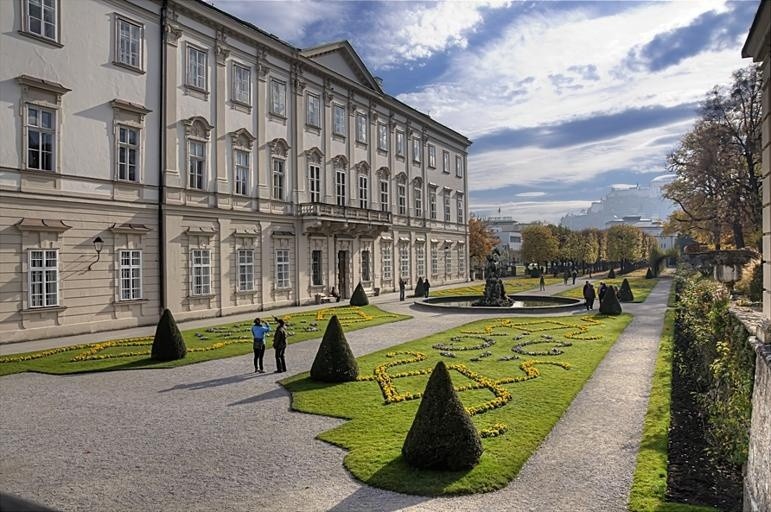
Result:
[254,367,288,374]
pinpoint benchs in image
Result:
[363,287,376,296]
[317,292,337,302]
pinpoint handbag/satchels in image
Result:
[253,341,265,351]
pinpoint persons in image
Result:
[332,287,342,302]
[269,313,289,373]
[399,277,407,301]
[564,270,569,286]
[584,280,621,312]
[423,278,431,299]
[252,317,272,373]
[540,275,546,292]
[573,272,578,285]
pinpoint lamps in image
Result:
[85,237,107,273]
